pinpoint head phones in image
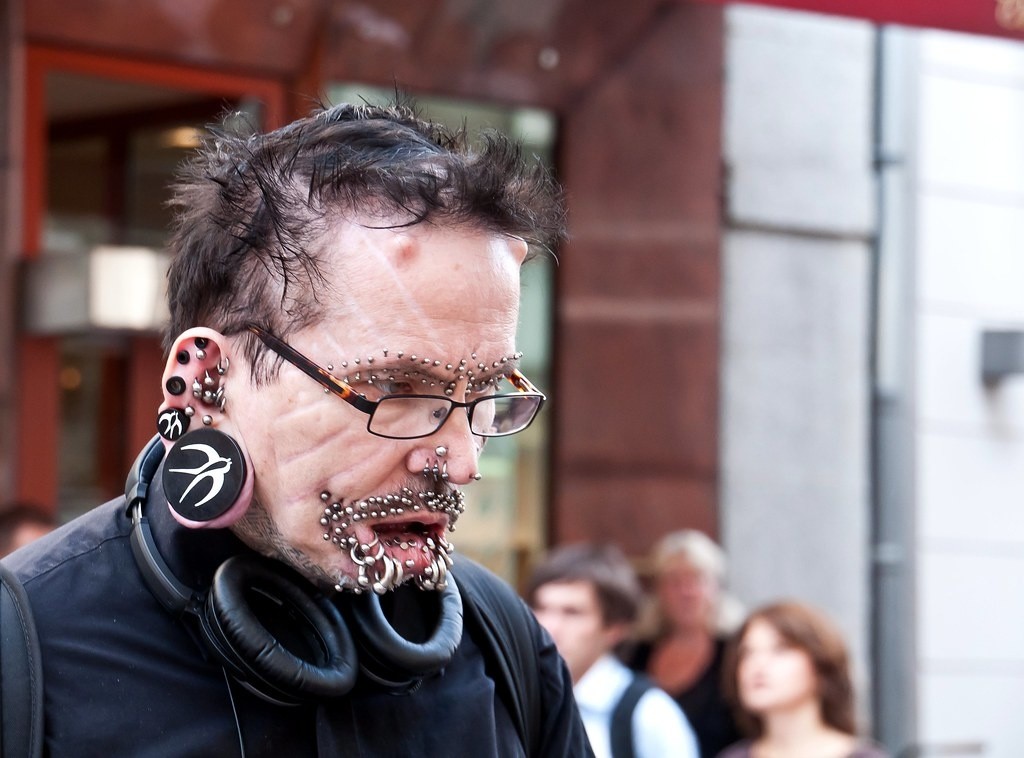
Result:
[123,430,464,711]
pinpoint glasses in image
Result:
[220,317,546,441]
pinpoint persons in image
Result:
[616,528,766,755]
[4,503,63,564]
[0,106,594,758]
[717,603,888,755]
[523,547,699,755]
